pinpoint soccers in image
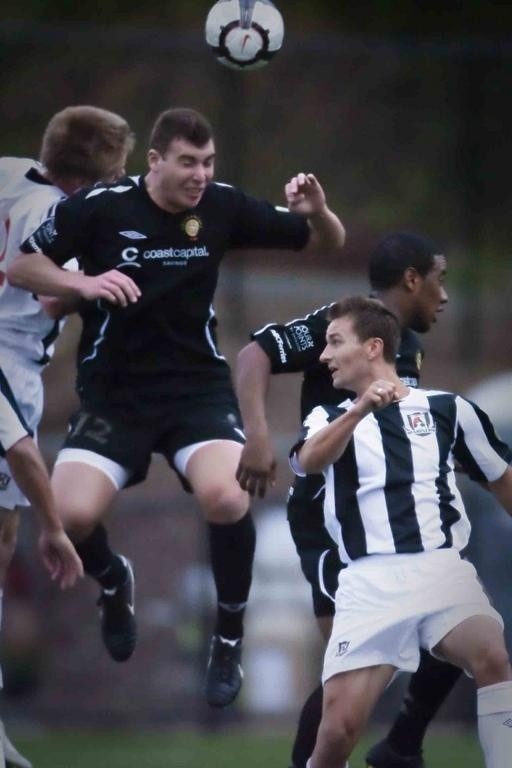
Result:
[205,0,284,71]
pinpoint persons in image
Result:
[0,363,90,767]
[285,293,511,767]
[225,220,471,767]
[3,101,348,714]
[2,100,137,584]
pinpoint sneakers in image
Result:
[99,554,138,661]
[365,736,428,767]
[202,632,243,706]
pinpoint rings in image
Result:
[375,387,383,394]
